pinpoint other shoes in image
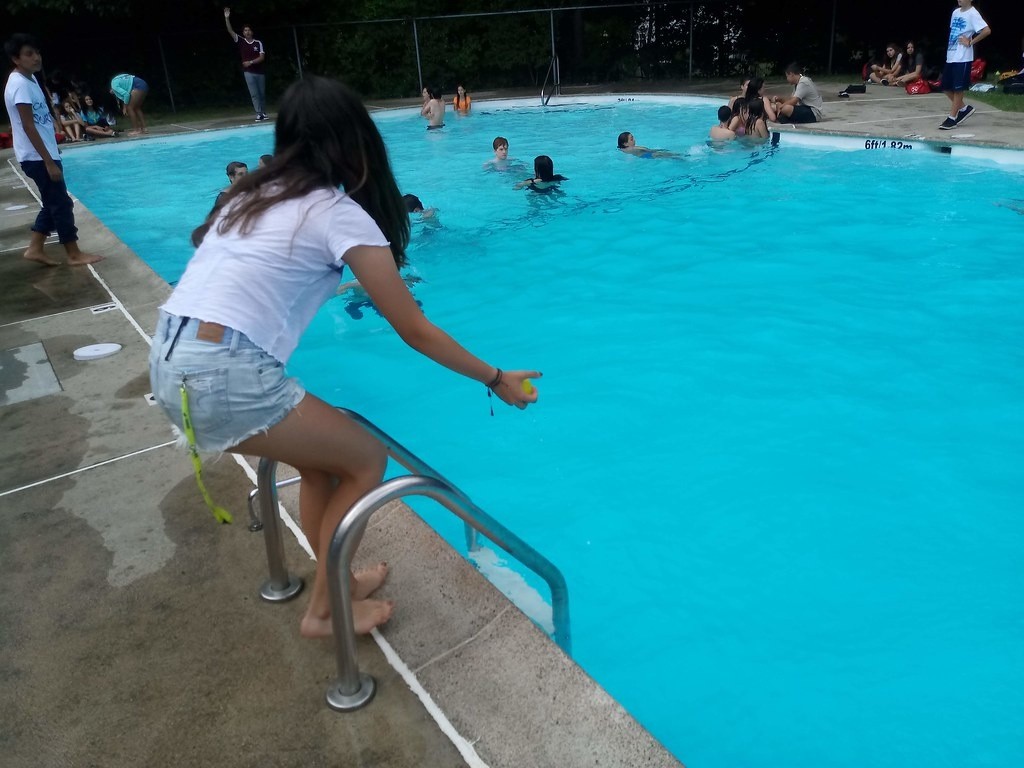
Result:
[82,133,96,141]
[838,92,849,97]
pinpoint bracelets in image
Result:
[250,61,253,65]
[486,368,503,416]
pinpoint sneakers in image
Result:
[262,112,269,120]
[955,106,975,124]
[939,114,960,129]
[254,114,263,122]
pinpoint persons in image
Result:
[493,136,509,160]
[42,68,119,143]
[452,84,471,110]
[617,132,647,149]
[225,161,248,184]
[710,62,823,139]
[149,76,543,641]
[259,154,273,169]
[868,39,924,86]
[525,155,569,182]
[4,32,105,264]
[224,7,269,122]
[420,86,445,125]
[109,73,149,134]
[939,0,991,130]
[403,194,424,212]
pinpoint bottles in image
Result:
[995,71,1000,82]
